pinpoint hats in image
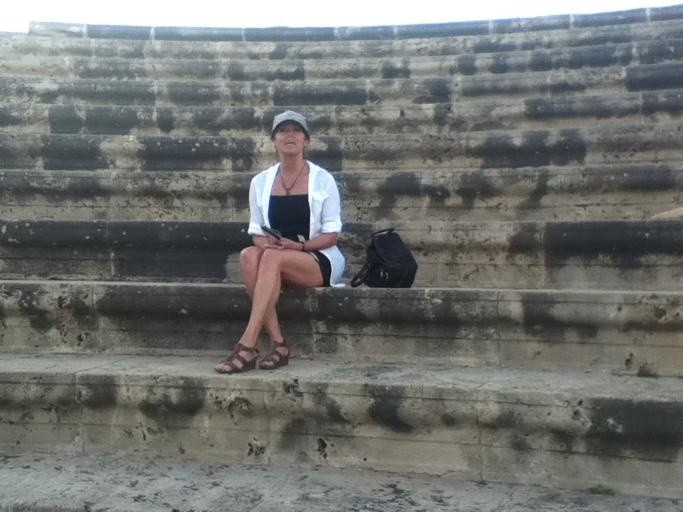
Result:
[270,109,311,141]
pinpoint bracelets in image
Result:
[299,241,305,250]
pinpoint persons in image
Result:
[214,110,347,374]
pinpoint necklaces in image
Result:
[277,163,304,195]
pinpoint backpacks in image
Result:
[349,227,418,288]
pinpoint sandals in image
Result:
[258,337,291,370]
[214,341,261,375]
